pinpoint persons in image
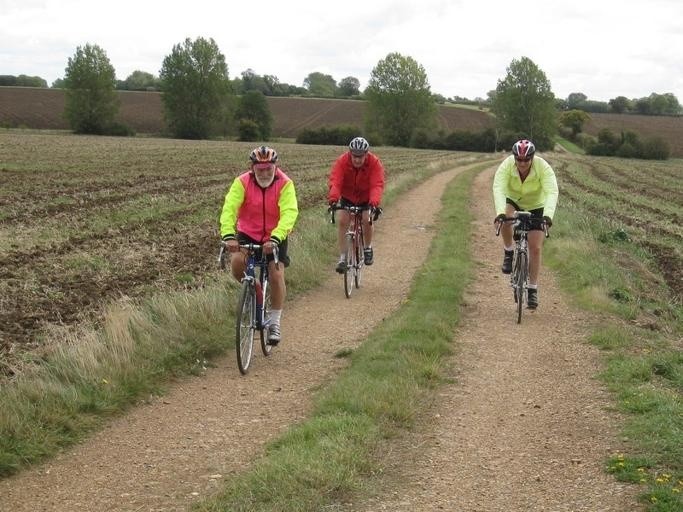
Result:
[217,145,300,346]
[326,136,386,275]
[491,137,560,311]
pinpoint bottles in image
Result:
[253,279,263,306]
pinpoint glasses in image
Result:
[515,157,532,162]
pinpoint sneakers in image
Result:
[269,323,281,342]
[503,250,514,273]
[364,246,374,265]
[336,261,346,273]
[528,288,538,306]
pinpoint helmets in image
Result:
[512,140,535,158]
[349,137,369,156]
[248,147,278,169]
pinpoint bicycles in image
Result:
[216,239,280,375]
[493,216,550,325]
[326,203,381,299]
